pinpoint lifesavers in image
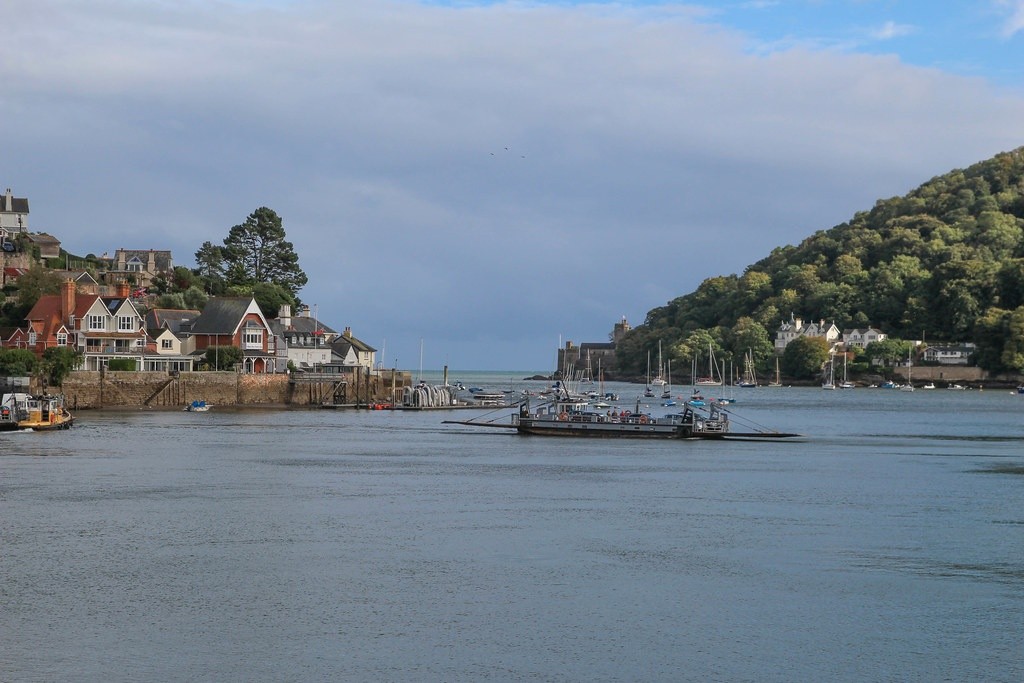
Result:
[625,410,631,416]
[680,428,690,438]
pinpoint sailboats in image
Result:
[439,338,968,399]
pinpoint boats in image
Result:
[441,402,805,439]
[0,386,75,433]
[183,400,214,411]
